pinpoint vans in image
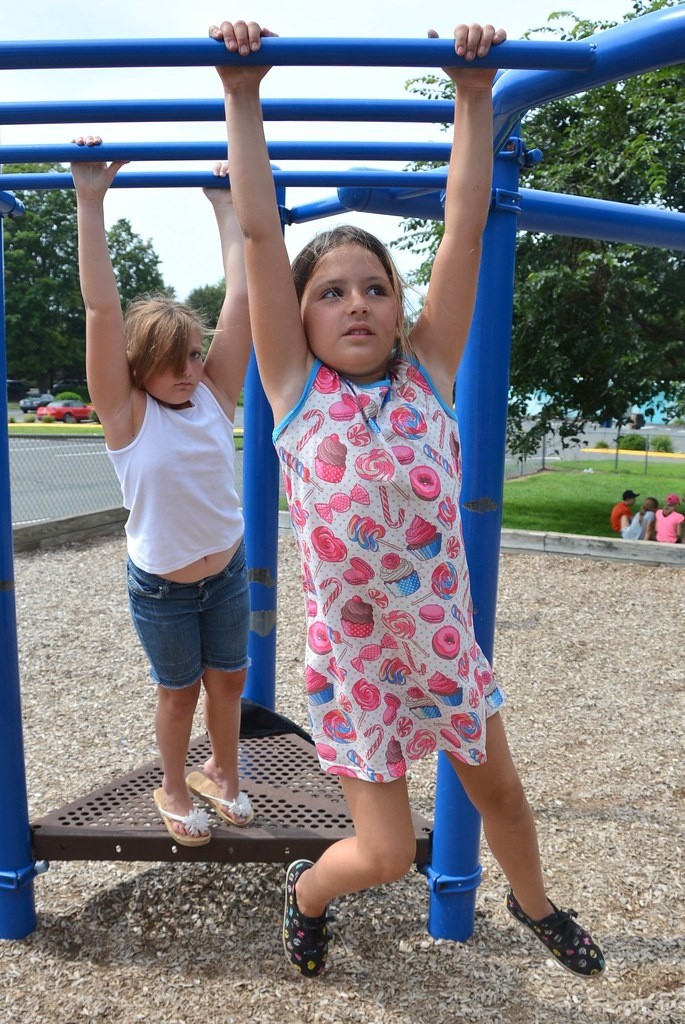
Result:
[52,378,87,394]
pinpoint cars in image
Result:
[36,399,94,424]
[19,392,55,413]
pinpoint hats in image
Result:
[623,490,640,500]
[666,494,679,503]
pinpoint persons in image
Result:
[68,137,255,845]
[611,489,685,545]
[211,21,605,981]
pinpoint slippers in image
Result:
[154,786,211,846]
[186,770,254,826]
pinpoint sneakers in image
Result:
[282,858,335,978]
[504,886,607,978]
